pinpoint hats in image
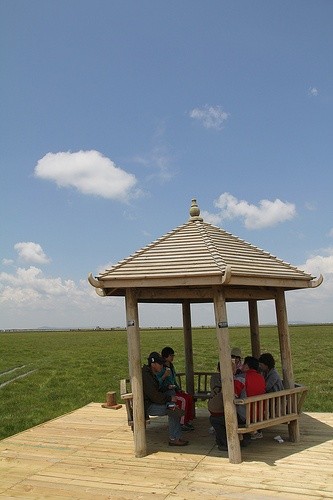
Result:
[231,347,243,356]
[148,356,166,365]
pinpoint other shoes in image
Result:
[218,445,228,451]
[184,423,195,430]
[209,426,215,433]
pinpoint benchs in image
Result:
[119,372,307,435]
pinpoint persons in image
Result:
[142,346,195,446]
[208,347,285,450]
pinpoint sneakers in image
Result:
[166,406,183,416]
[169,438,188,446]
[250,433,263,440]
